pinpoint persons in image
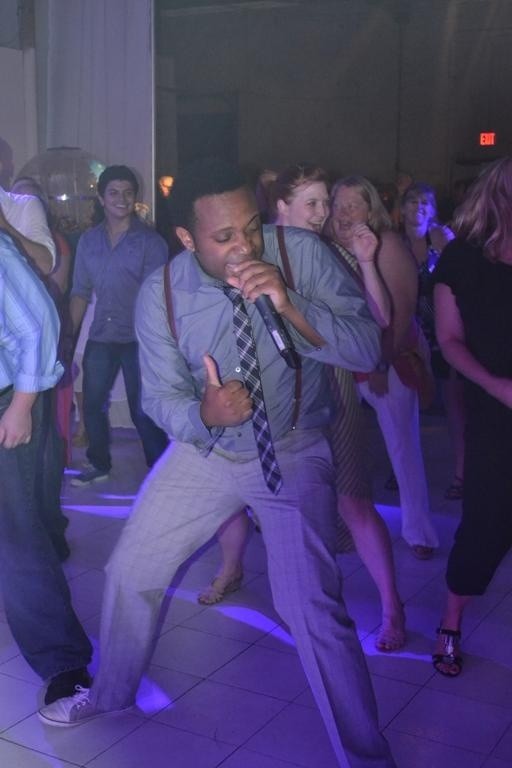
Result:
[1,140,512,767]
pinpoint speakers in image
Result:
[175,90,240,184]
[0,0,36,50]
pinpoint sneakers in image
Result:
[71,460,111,486]
[36,683,135,729]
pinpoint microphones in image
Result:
[255,293,302,370]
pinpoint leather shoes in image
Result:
[45,665,92,704]
[53,531,69,563]
[59,513,71,536]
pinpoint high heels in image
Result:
[431,623,464,677]
[373,603,408,653]
[197,566,244,607]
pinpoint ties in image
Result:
[222,283,284,496]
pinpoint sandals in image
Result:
[409,543,434,561]
[446,471,466,499]
[385,470,400,491]
